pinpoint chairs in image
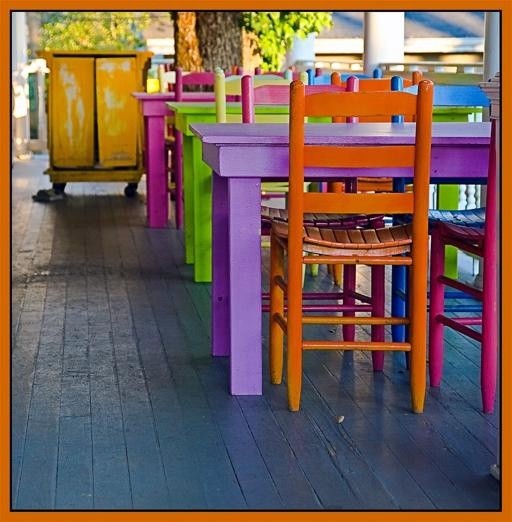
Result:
[305,67,383,193]
[214,71,319,277]
[269,80,433,414]
[157,64,192,222]
[174,66,261,229]
[241,75,385,372]
[389,76,493,342]
[431,118,500,415]
[327,71,424,286]
[254,67,328,199]
[218,68,292,80]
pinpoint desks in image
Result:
[190,123,492,395]
[165,101,485,283]
[129,92,240,230]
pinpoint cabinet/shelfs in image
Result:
[33,48,154,197]
